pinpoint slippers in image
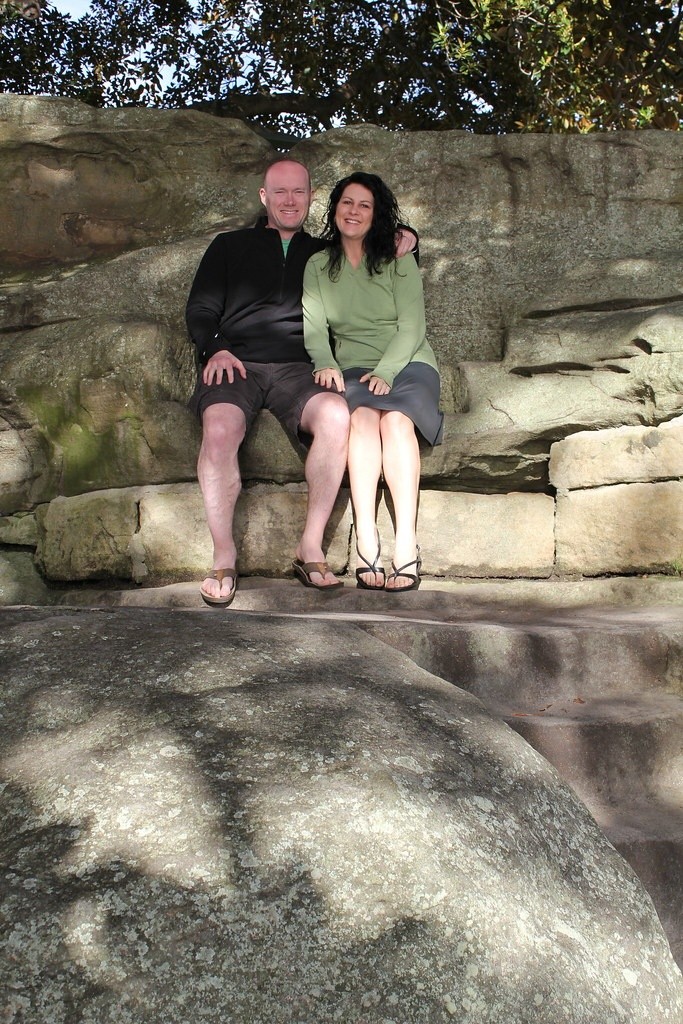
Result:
[355,540,385,590]
[200,568,237,603]
[291,557,344,589]
[385,544,421,592]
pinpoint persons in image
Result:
[302,158,441,593]
[186,159,418,603]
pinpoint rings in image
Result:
[387,387,389,388]
